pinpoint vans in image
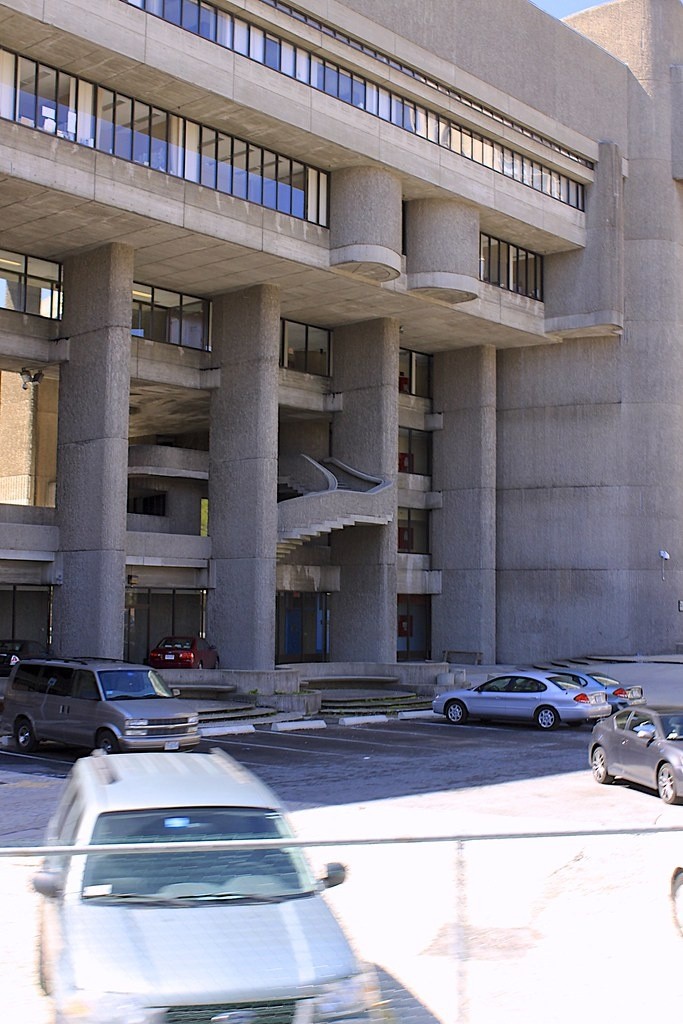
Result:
[4,657,202,756]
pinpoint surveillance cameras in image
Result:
[663,553,670,560]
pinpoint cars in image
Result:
[0,638,51,675]
[150,637,220,669]
[588,703,683,805]
[517,671,648,713]
[432,671,612,732]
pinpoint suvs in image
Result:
[31,748,385,1023]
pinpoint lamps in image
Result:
[20,367,44,390]
[128,575,139,587]
